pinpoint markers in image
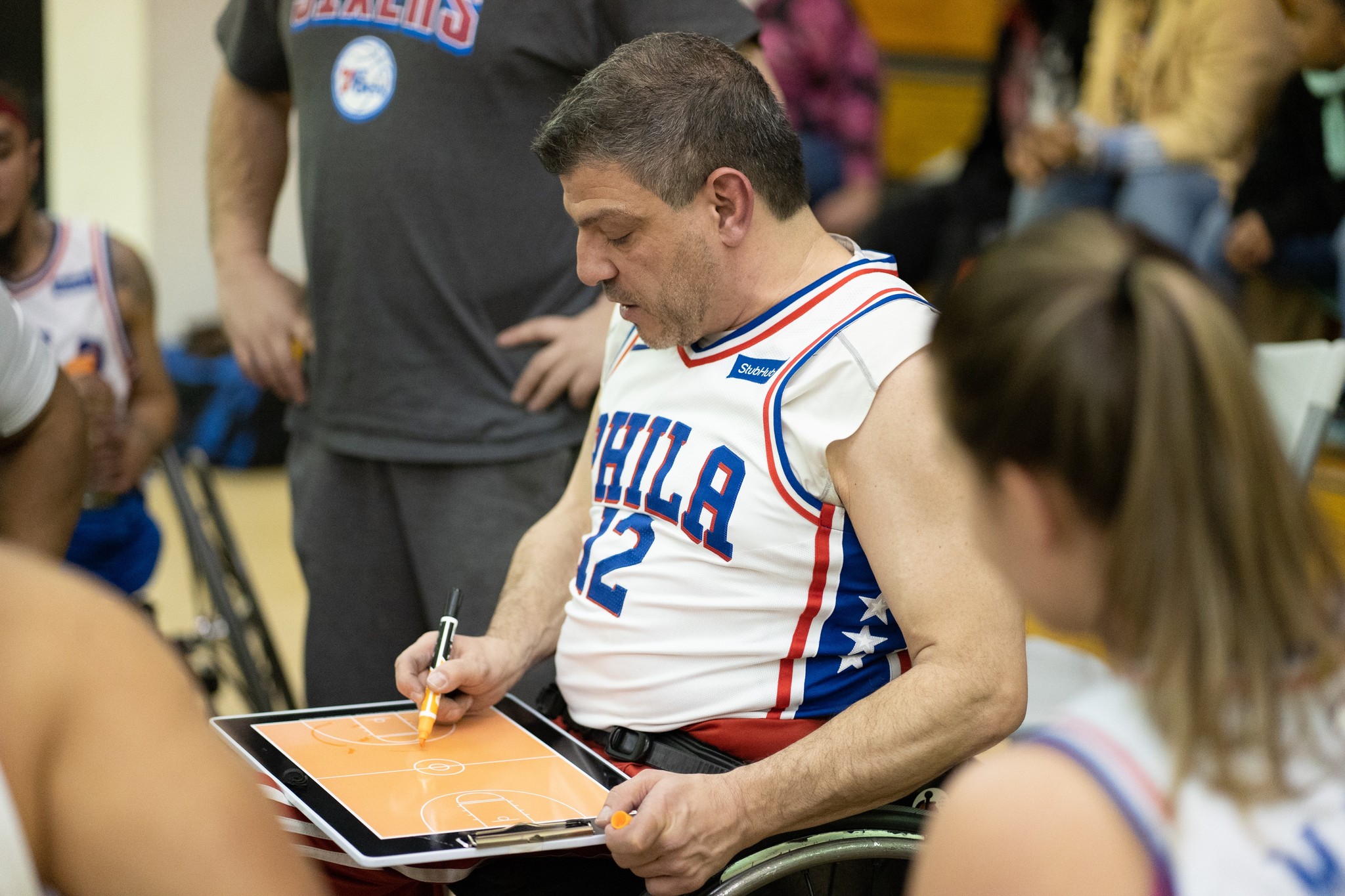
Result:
[417,587,465,747]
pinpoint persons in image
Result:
[889,205,1345,896]
[0,0,1345,896]
[198,0,786,730]
[386,29,1033,895]
